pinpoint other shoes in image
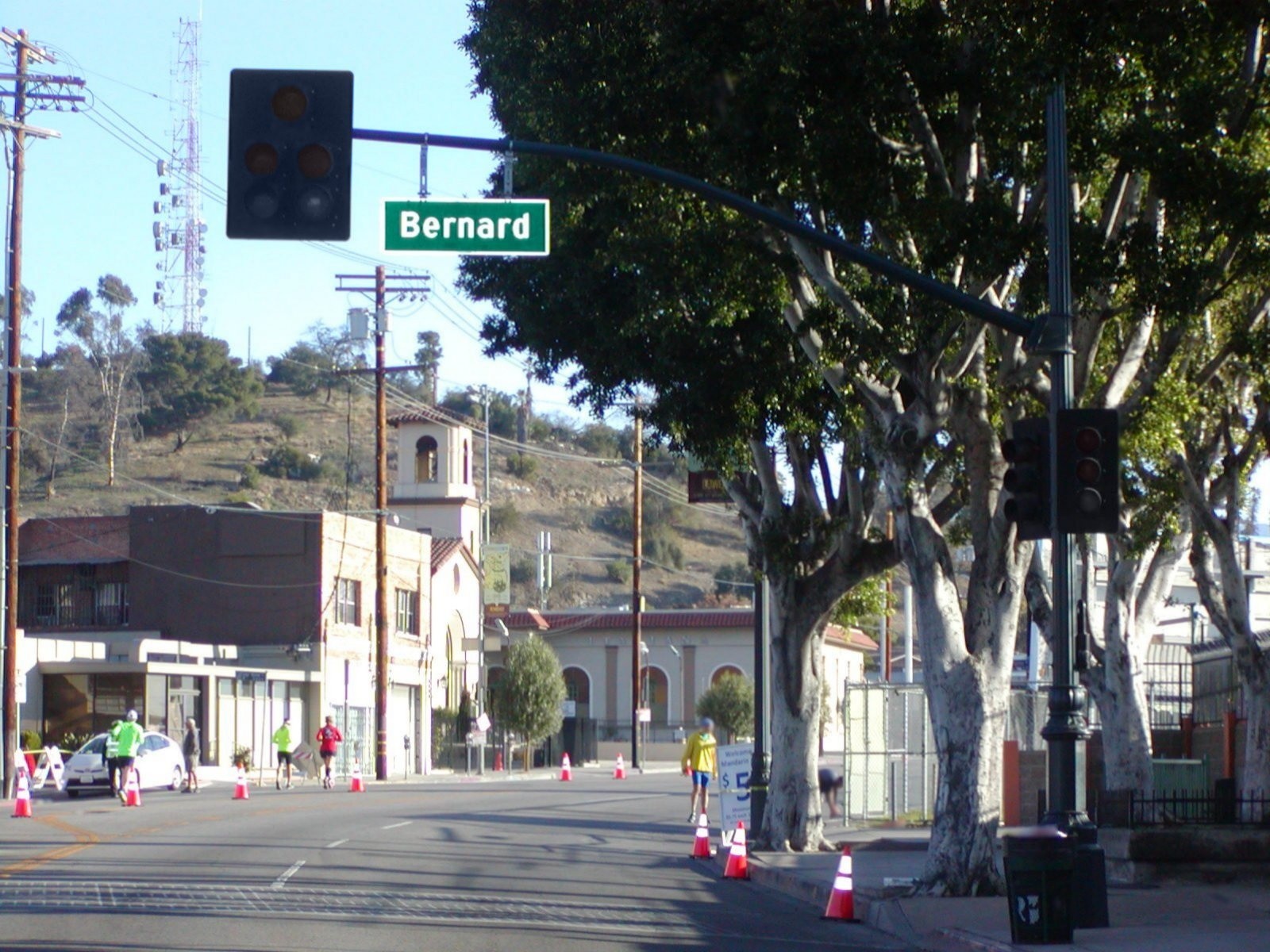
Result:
[110,792,119,798]
[286,784,294,789]
[688,814,695,822]
[324,779,327,789]
[118,789,127,802]
[327,780,334,788]
[193,787,200,792]
[829,813,844,818]
[181,787,192,792]
[277,780,281,790]
[706,815,710,824]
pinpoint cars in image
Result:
[62,730,186,797]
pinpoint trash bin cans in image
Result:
[1001,831,1074,945]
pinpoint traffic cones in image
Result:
[493,752,503,771]
[691,809,714,860]
[681,758,692,776]
[560,753,573,782]
[12,769,33,818]
[233,763,250,800]
[822,846,861,924]
[722,821,749,879]
[346,756,368,793]
[613,753,627,779]
[123,766,144,806]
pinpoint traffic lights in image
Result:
[1000,413,1051,540]
[227,69,354,239]
[1059,408,1119,531]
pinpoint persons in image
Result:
[316,715,343,790]
[180,717,201,795]
[818,768,845,820]
[270,717,295,790]
[100,720,125,799]
[680,717,719,824]
[109,709,145,804]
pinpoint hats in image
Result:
[127,709,138,718]
[702,718,713,725]
